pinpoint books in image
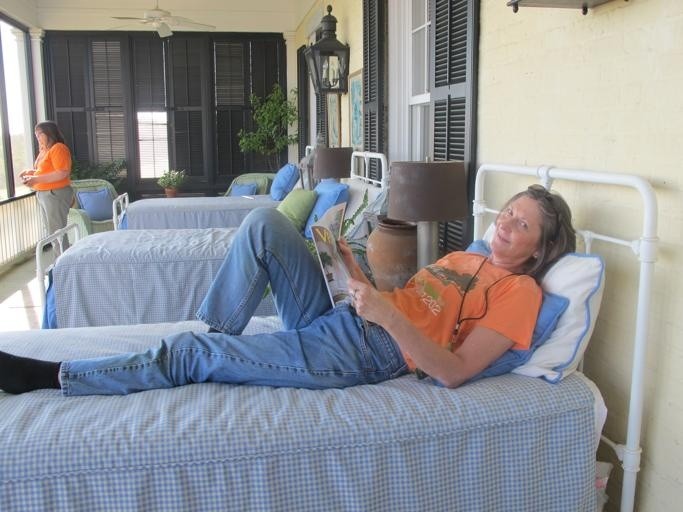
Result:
[307,201,354,308]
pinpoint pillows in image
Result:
[229,183,257,196]
[482,223,605,383]
[429,238,570,388]
[270,162,300,201]
[305,177,349,239]
[75,187,118,221]
[275,188,317,232]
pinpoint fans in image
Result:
[110,1,216,40]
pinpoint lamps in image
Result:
[303,4,349,95]
[312,147,352,178]
[389,161,468,273]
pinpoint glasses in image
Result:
[527,184,557,212]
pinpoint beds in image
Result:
[113,145,314,230]
[35,151,387,330]
[1,164,656,511]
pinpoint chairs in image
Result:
[224,173,269,195]
[69,179,122,234]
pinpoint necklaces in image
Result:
[449,256,523,353]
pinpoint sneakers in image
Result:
[45,264,55,275]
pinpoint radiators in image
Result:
[1,191,46,276]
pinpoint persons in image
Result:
[19,120,72,260]
[0,184,577,397]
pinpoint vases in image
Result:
[364,222,418,290]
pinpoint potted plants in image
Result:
[157,168,185,198]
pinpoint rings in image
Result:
[350,289,355,297]
